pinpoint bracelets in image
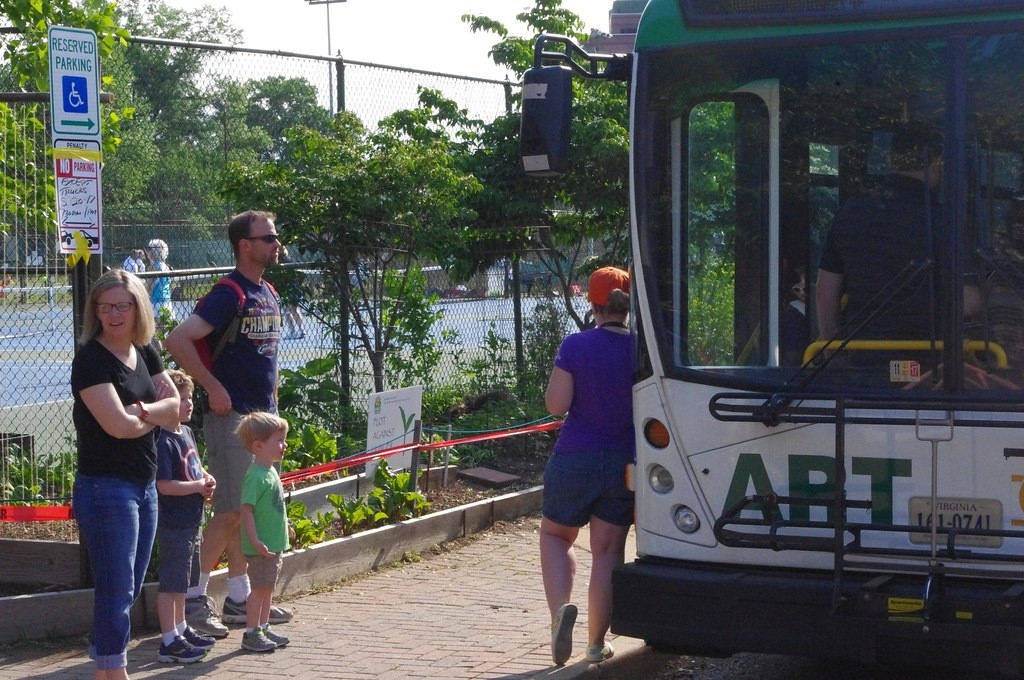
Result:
[135,400,149,421]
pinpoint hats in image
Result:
[587,266,629,307]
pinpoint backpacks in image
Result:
[188,277,284,375]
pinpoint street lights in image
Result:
[213,98,235,268]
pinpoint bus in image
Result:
[519,1,1024,667]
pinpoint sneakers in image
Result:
[551,604,578,665]
[182,625,216,651]
[184,594,229,637]
[241,626,277,652]
[262,622,290,647]
[158,634,208,663]
[222,592,294,622]
[585,640,614,662]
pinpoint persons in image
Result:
[71,268,181,680]
[155,368,216,664]
[162,209,293,637]
[232,408,296,652]
[779,262,806,366]
[123,249,145,283]
[278,245,306,340]
[144,239,176,361]
[815,121,983,390]
[540,266,637,664]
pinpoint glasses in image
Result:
[94,299,136,314]
[236,233,279,244]
